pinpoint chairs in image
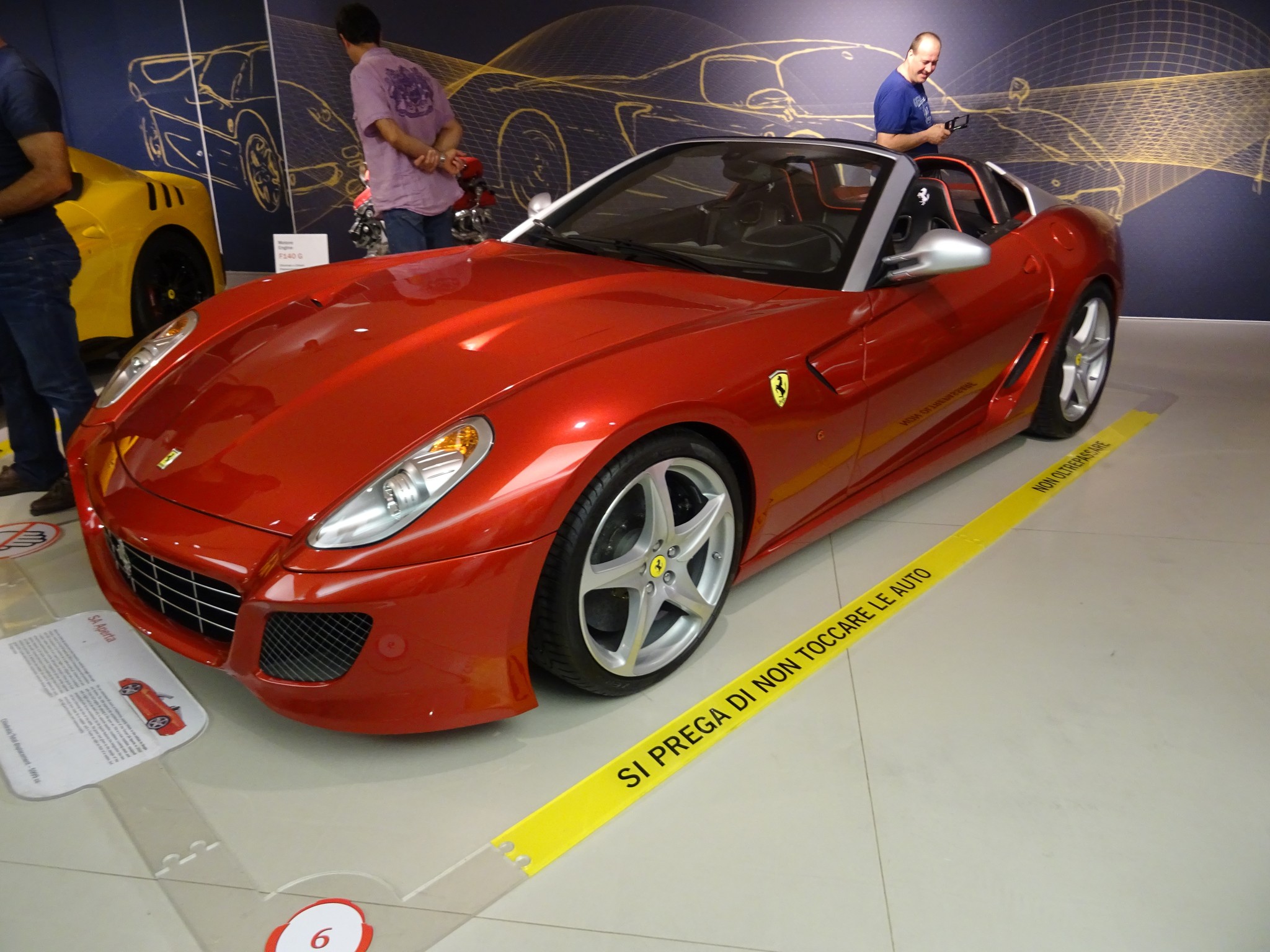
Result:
[700,163,806,247]
[891,176,978,254]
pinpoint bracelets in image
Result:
[440,154,445,166]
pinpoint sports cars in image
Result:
[65,134,1125,736]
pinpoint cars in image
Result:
[53,146,227,373]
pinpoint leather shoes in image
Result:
[0,463,38,496]
[29,472,76,516]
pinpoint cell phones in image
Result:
[948,124,968,131]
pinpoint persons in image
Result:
[870,32,951,186]
[0,39,97,514]
[335,3,466,254]
